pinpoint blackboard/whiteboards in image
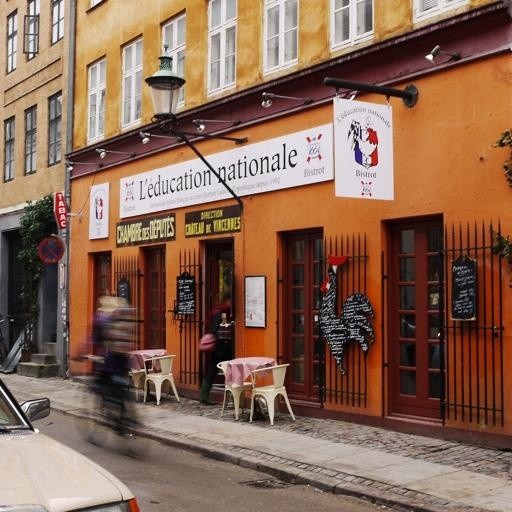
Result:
[452,256,477,321]
[177,277,195,315]
[117,281,129,297]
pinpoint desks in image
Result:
[230,357,276,421]
[128,350,167,401]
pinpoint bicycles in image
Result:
[71,353,151,459]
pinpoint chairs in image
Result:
[216,360,264,421]
[249,363,296,426]
[143,355,180,406]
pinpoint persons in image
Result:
[199,292,233,404]
[88,293,132,423]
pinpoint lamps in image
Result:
[139,131,183,145]
[64,162,103,173]
[145,43,245,208]
[192,118,240,133]
[260,92,311,108]
[95,148,136,159]
[425,45,462,64]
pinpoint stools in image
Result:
[128,369,154,401]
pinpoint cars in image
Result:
[0,377,141,511]
